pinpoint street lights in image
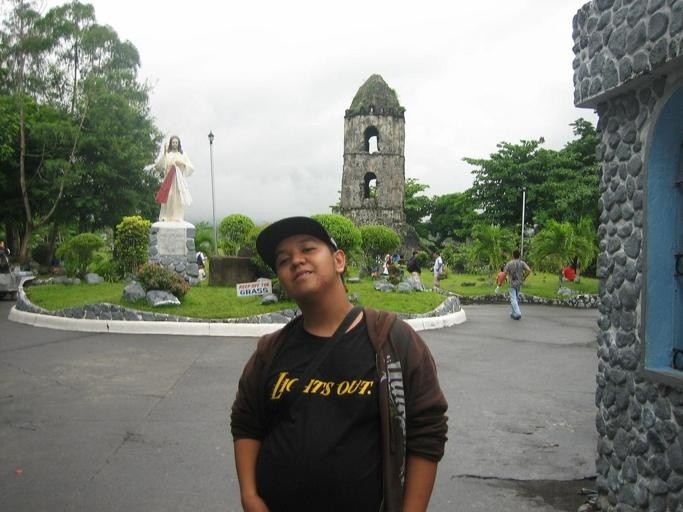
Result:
[520,185,527,257]
[207,129,218,255]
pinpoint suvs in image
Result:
[0,248,36,302]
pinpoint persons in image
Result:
[198,263,205,281]
[499,250,531,320]
[559,263,576,283]
[369,250,406,279]
[493,268,506,294]
[154,134,193,221]
[406,251,422,290]
[230,217,447,511]
[0,240,10,255]
[432,251,444,288]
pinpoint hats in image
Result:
[255,215,341,274]
